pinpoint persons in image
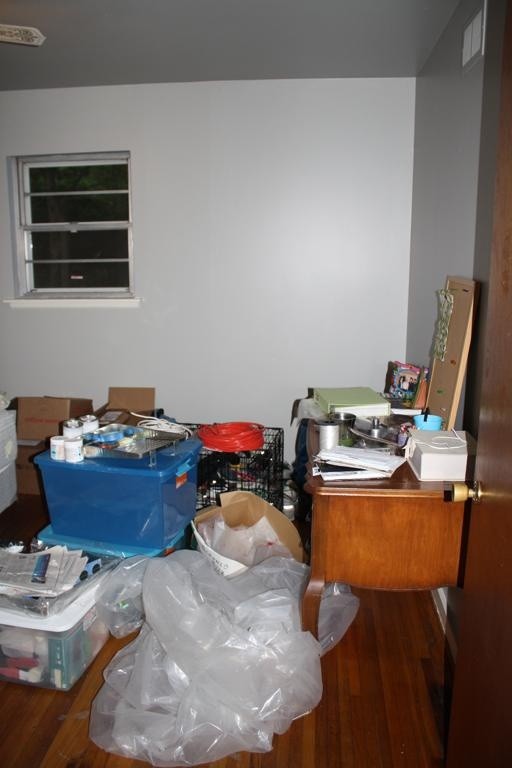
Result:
[399,376,417,391]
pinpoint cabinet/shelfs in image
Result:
[303,413,466,589]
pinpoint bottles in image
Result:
[50,415,99,463]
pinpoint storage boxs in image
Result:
[0,386,203,693]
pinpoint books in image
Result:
[312,445,407,481]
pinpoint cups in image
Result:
[413,415,442,431]
[318,412,356,450]
